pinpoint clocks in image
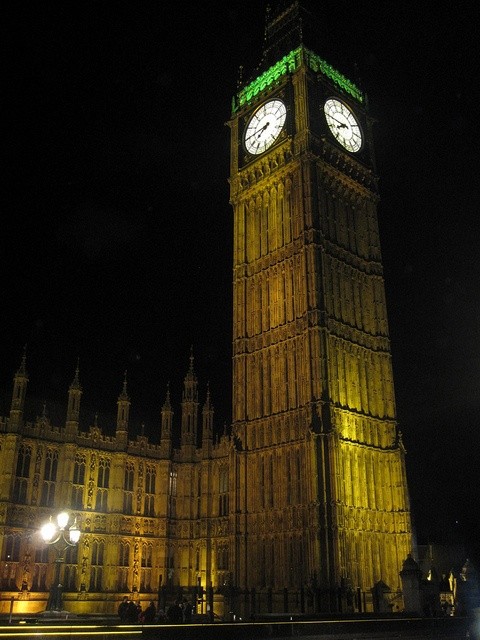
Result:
[320,96,368,154]
[238,84,296,169]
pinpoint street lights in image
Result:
[40,510,81,611]
